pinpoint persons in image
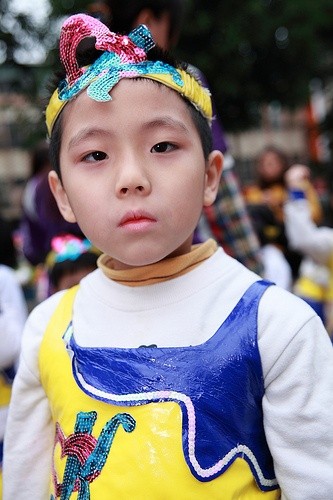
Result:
[47,254,100,294]
[0,42,333,500]
[0,262,27,468]
[244,146,332,342]
